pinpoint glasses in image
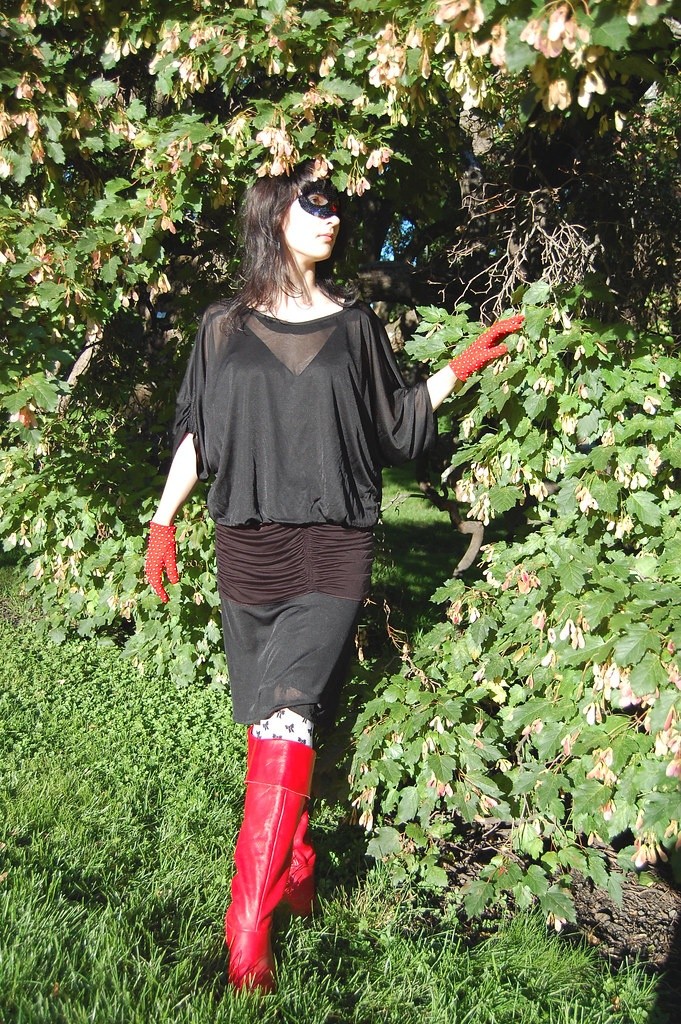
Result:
[297,178,339,219]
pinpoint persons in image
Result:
[145,160,525,998]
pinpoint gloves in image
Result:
[449,316,524,382]
[146,522,178,603]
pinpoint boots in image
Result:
[225,738,317,996]
[247,725,315,919]
